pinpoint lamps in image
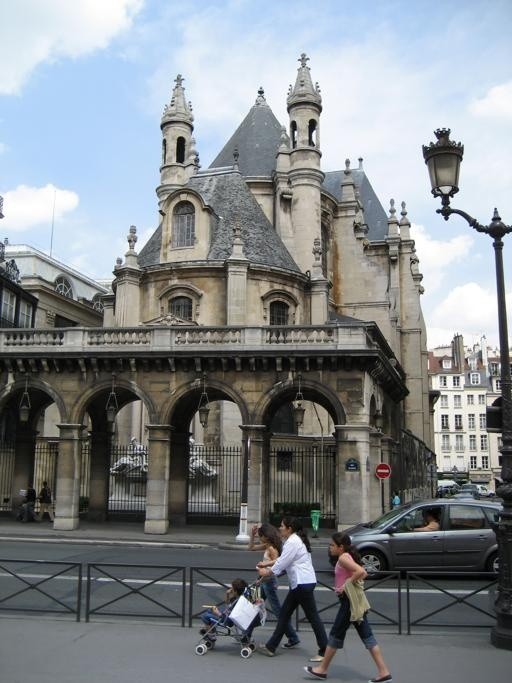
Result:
[105,370,118,424]
[198,371,210,427]
[291,375,306,426]
[18,376,32,427]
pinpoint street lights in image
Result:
[421,124,511,652]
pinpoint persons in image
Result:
[302,532,393,683]
[201,577,249,642]
[22,482,43,523]
[403,508,441,533]
[126,437,148,473]
[36,480,55,522]
[254,514,328,663]
[390,488,401,510]
[232,521,301,649]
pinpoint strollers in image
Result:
[195,566,266,659]
[14,503,35,523]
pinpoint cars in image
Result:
[327,484,504,581]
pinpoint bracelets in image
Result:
[411,527,414,532]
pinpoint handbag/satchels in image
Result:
[228,584,267,631]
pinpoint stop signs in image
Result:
[375,462,392,480]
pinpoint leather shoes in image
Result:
[310,654,324,661]
[258,640,274,656]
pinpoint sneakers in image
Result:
[304,665,326,679]
[368,674,393,682]
[281,639,300,648]
